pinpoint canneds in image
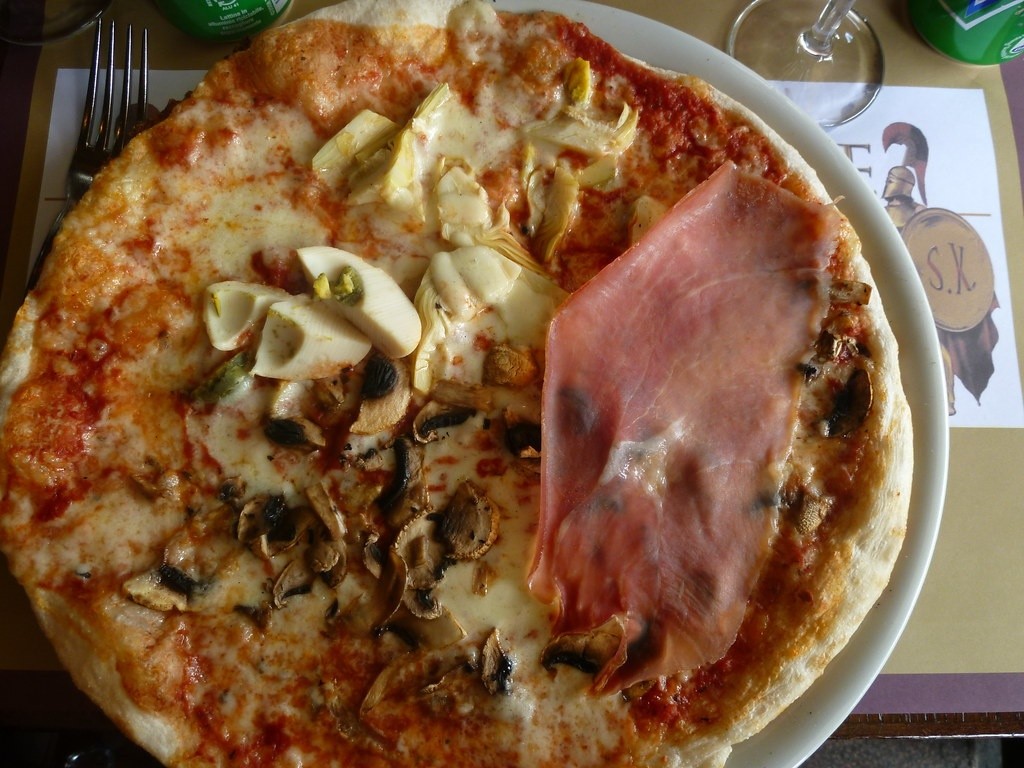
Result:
[154,0,296,47]
[908,0,1024,68]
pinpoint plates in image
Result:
[497,0,952,768]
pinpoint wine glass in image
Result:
[727,0,885,126]
[0,0,109,46]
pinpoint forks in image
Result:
[21,17,148,298]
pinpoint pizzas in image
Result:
[0,0,913,768]
